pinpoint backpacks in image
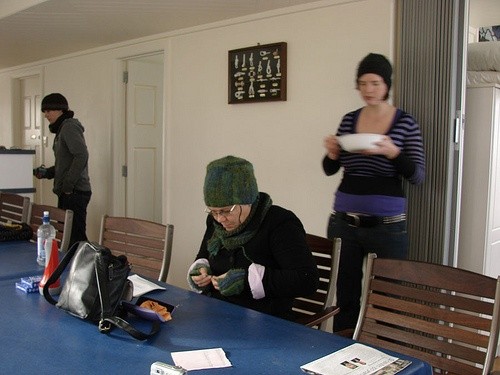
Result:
[42,240,160,340]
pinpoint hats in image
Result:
[203,155,258,206]
[40,91,69,113]
[355,53,393,90]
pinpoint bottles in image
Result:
[37,211,56,266]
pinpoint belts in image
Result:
[330,209,407,228]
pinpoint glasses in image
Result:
[204,205,236,216]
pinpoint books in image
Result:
[299,342,412,375]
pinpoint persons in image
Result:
[322,52,425,344]
[185,156,320,320]
[32,93,91,254]
[340,358,366,369]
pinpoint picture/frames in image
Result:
[227,41,288,105]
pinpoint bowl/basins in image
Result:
[336,133,389,153]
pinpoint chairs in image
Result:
[27,202,73,252]
[99,213,175,283]
[353,253,500,375]
[288,233,342,331]
[0,191,30,228]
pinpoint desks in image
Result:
[0,242,432,375]
